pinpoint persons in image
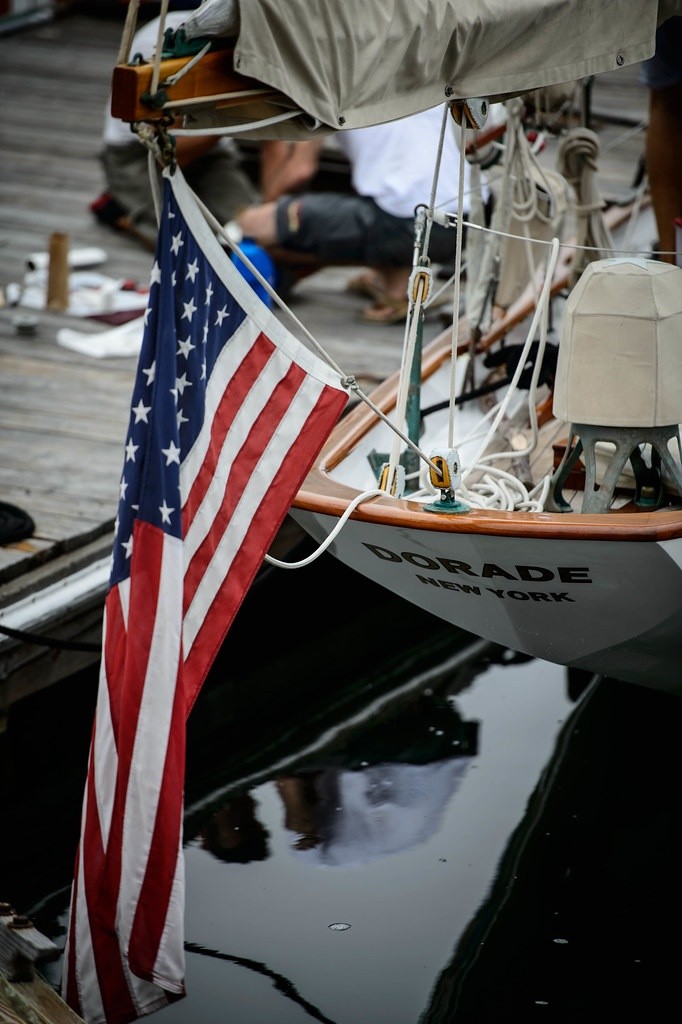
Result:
[226,104,492,323]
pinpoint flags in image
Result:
[56,167,348,1024]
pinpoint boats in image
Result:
[93,2,682,707]
